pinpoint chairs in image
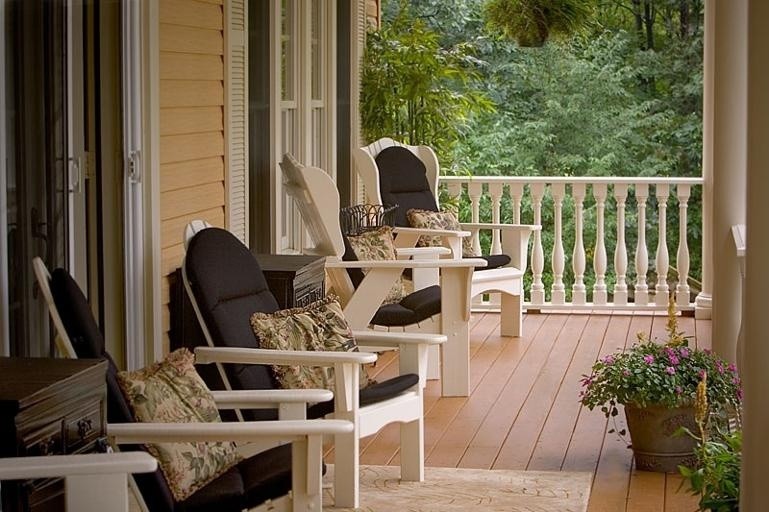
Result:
[352,135,543,336]
[33,257,357,511]
[171,227,446,508]
[0,452,158,512]
[277,154,488,398]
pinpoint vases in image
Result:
[622,398,702,472]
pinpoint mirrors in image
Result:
[183,218,216,254]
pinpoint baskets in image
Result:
[340,203,399,237]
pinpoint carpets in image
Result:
[241,465,594,512]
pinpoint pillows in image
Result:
[117,346,244,499]
[251,299,373,397]
[407,209,464,257]
[344,227,403,306]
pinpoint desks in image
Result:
[0,350,109,512]
[164,254,327,352]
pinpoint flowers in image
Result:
[579,287,741,452]
